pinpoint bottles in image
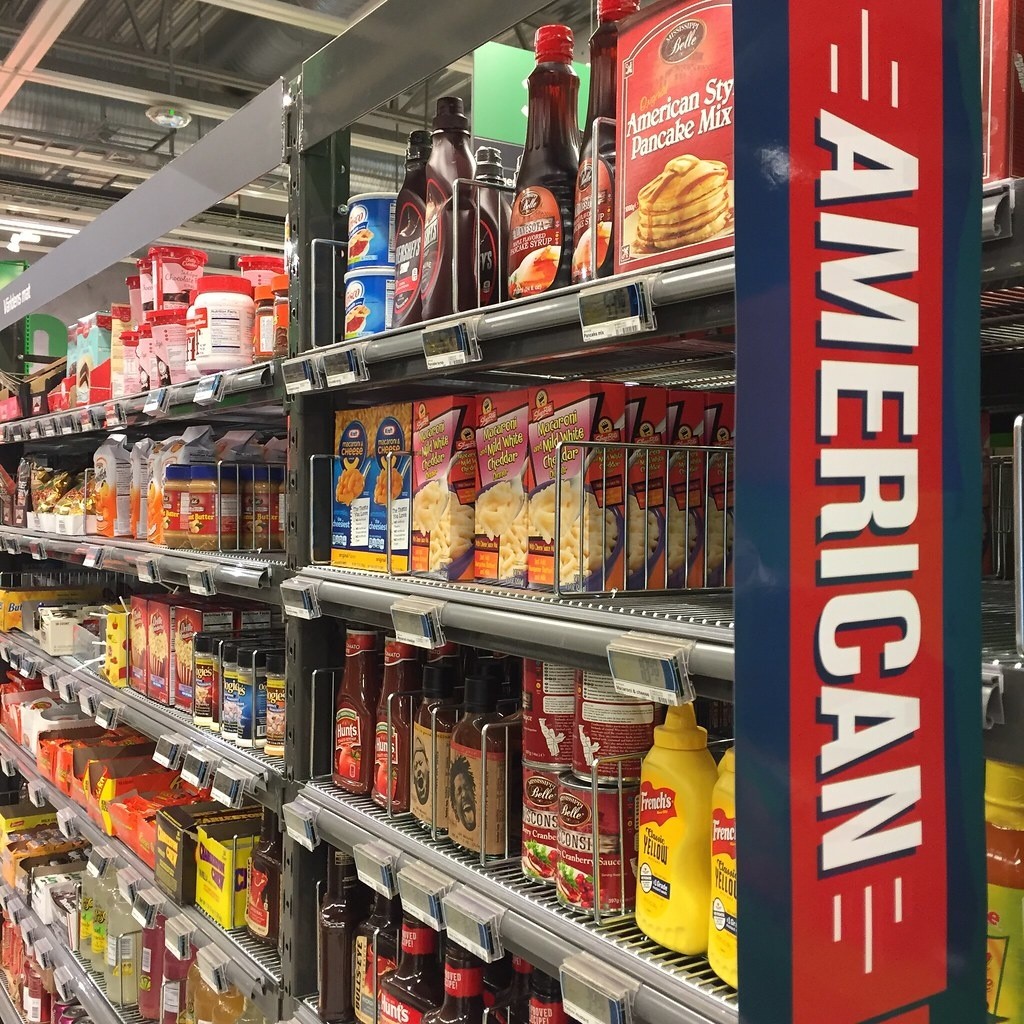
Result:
[331,622,523,863]
[312,844,576,1024]
[633,696,737,990]
[391,96,512,328]
[570,0,643,284]
[246,807,283,947]
[506,24,581,301]
[194,628,285,756]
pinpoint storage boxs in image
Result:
[329,381,734,597]
[49,882,81,952]
[1,689,59,745]
[105,787,189,853]
[0,802,58,857]
[69,742,157,809]
[54,736,148,796]
[1,837,90,888]
[195,817,262,931]
[0,682,43,727]
[153,801,267,907]
[0,302,283,714]
[87,759,182,837]
[613,0,734,274]
[134,801,198,870]
[13,850,89,907]
[31,860,87,925]
[35,724,138,782]
[30,707,95,756]
[20,698,80,752]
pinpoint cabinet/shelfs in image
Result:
[0,0,990,1024]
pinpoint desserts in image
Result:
[638,154,730,250]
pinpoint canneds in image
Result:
[53,993,96,1024]
[181,190,402,376]
[521,656,664,916]
[191,629,287,756]
